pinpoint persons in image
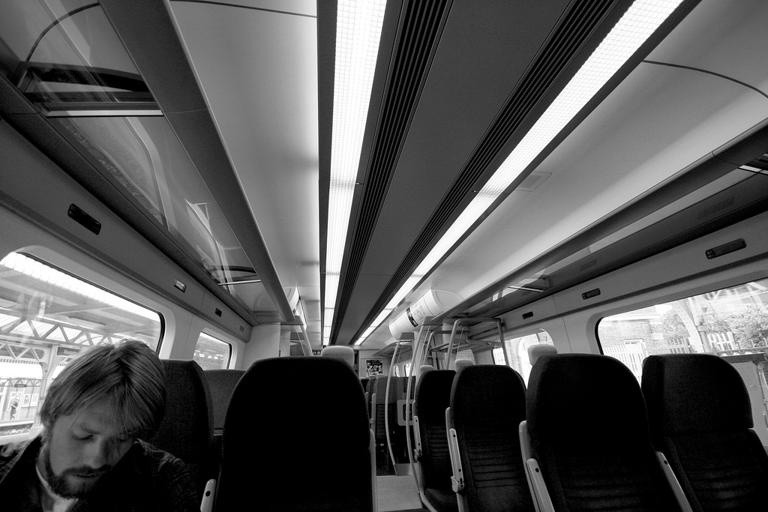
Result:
[1,337,193,511]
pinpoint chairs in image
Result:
[444,364,540,512]
[215,354,381,512]
[203,368,247,483]
[409,368,458,512]
[360,375,417,476]
[518,351,686,512]
[639,352,768,512]
[158,358,214,512]
